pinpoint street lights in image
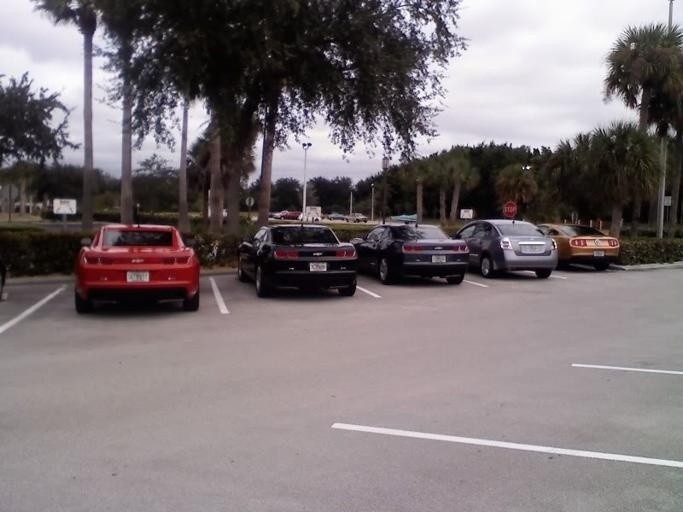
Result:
[655,123,678,240]
[301,142,312,218]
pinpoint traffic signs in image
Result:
[503,202,518,217]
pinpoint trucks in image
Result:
[298,206,322,222]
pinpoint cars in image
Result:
[75,223,201,313]
[349,222,470,286]
[328,213,345,221]
[237,222,359,298]
[273,210,301,220]
[345,213,368,223]
[536,222,622,270]
[455,219,559,278]
